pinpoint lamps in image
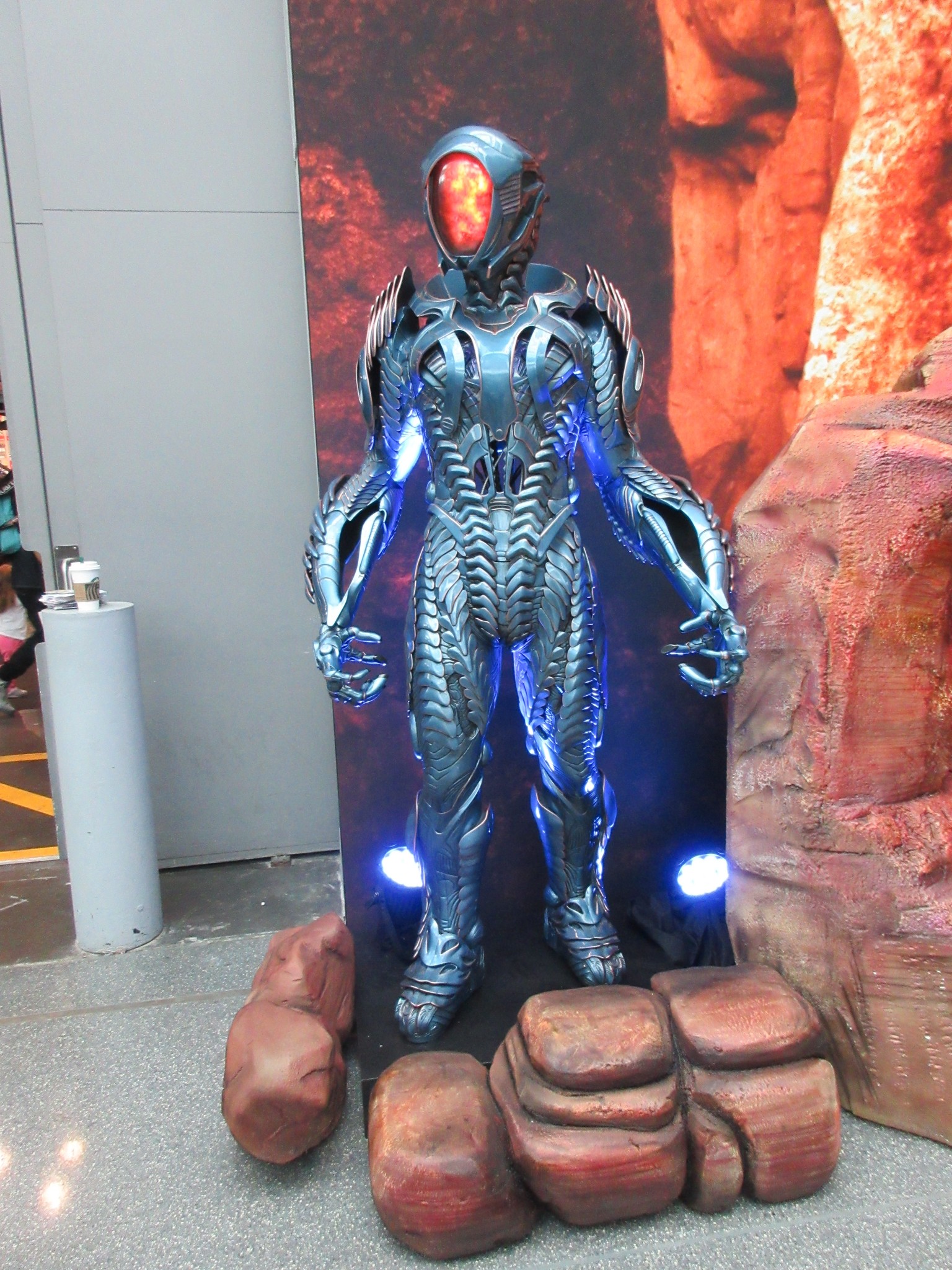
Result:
[626,843,734,966]
[360,840,426,965]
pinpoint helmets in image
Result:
[422,125,546,279]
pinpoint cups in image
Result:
[68,557,101,611]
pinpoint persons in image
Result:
[0,549,47,714]
[296,127,755,1046]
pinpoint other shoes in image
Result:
[6,686,28,699]
[0,679,16,715]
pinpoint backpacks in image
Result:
[0,480,22,562]
